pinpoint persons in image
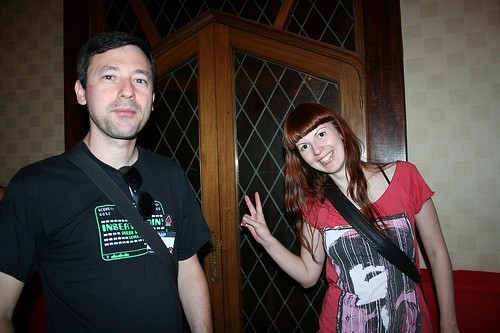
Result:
[0,31,213,333]
[241,104,458,333]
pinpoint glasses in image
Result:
[119,165,157,223]
